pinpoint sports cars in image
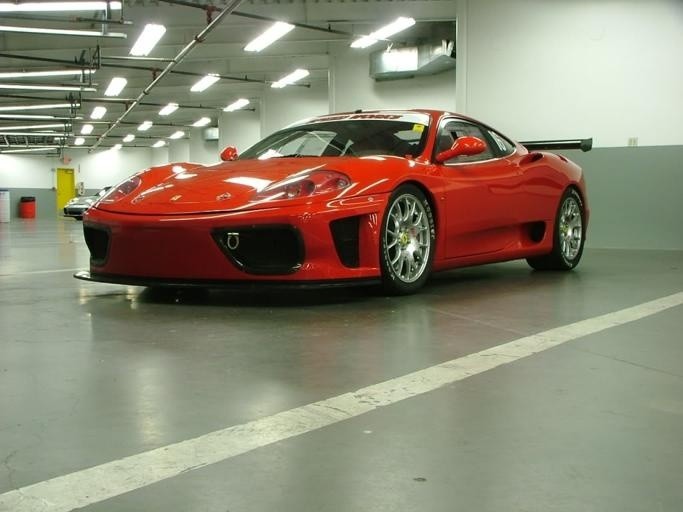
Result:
[71,105,593,301]
[61,185,113,221]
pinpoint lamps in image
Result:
[0,26,127,156]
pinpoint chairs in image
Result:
[343,128,409,156]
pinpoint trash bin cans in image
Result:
[20,196,35,218]
